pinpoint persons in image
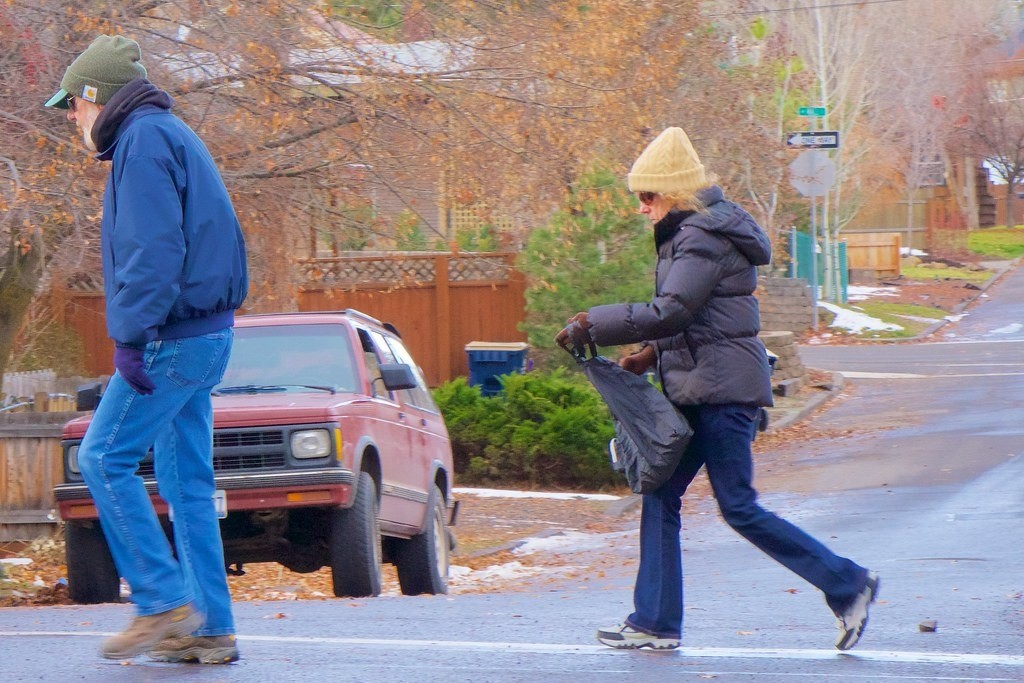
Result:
[46,34,250,663]
[554,126,878,650]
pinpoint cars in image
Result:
[54,308,461,607]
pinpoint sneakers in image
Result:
[98,602,205,660]
[834,570,880,650]
[148,636,239,664]
[596,622,681,649]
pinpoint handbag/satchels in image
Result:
[556,321,695,494]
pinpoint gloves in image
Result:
[113,347,157,396]
[620,345,652,376]
[554,311,589,349]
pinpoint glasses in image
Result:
[639,191,657,204]
[63,96,78,112]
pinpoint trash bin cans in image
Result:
[765,350,776,374]
[464,340,529,398]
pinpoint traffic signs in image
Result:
[785,131,840,151]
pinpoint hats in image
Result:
[60,33,148,106]
[45,89,68,110]
[628,127,707,192]
